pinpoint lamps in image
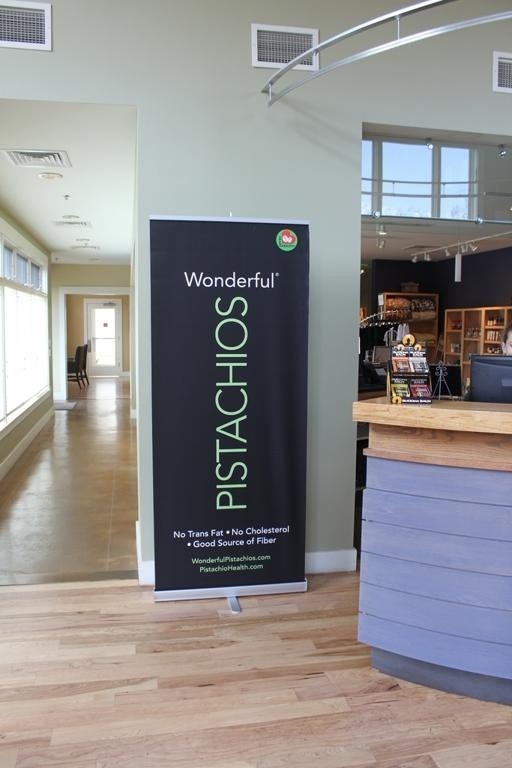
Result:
[411,254,418,263]
[444,247,452,257]
[455,240,462,283]
[469,241,479,252]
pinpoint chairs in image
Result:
[67,344,89,392]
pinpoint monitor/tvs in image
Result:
[467,354,511,402]
[372,345,391,365]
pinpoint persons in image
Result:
[501,325,512,357]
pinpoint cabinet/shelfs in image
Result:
[384,357,432,404]
[378,292,438,361]
[441,305,512,397]
